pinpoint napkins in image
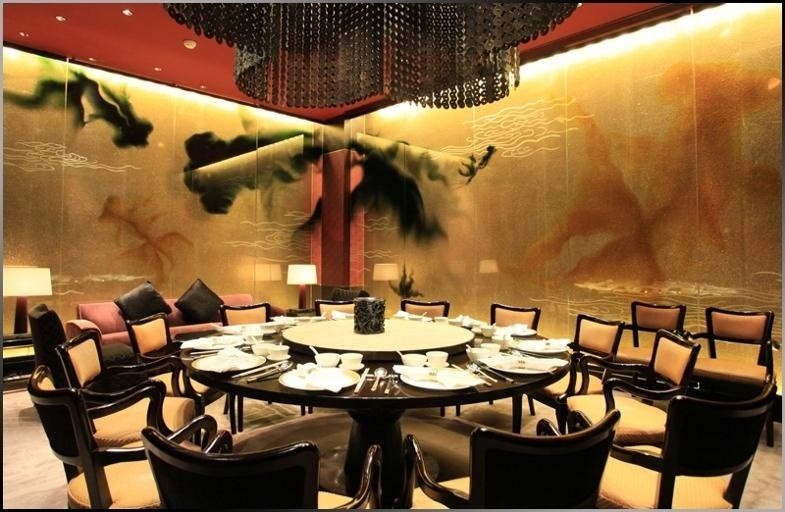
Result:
[505,323,529,337]
[533,336,572,350]
[482,355,563,370]
[215,345,252,373]
[394,365,466,391]
[297,360,342,393]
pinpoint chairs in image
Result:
[689,307,777,447]
[26,365,228,511]
[580,301,687,395]
[127,314,187,395]
[309,298,356,412]
[564,328,701,444]
[27,302,133,369]
[455,303,539,417]
[287,289,365,317]
[52,330,202,478]
[526,314,625,415]
[400,300,450,416]
[218,303,273,408]
[568,384,775,508]
[54,329,147,396]
[395,410,620,508]
[138,415,383,508]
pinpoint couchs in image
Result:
[64,293,287,346]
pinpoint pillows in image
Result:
[175,278,224,323]
[112,281,174,320]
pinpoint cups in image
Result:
[355,297,386,334]
[482,342,501,358]
[269,346,288,358]
[425,351,449,366]
[341,353,364,367]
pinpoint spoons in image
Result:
[246,362,293,383]
[466,361,498,383]
[371,367,387,392]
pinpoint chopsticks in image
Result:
[354,367,371,394]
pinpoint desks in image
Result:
[179,315,571,510]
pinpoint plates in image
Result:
[485,356,557,374]
[179,316,327,350]
[400,368,478,390]
[191,353,265,373]
[405,315,571,353]
[268,356,291,362]
[278,367,361,392]
[339,364,364,372]
[424,362,449,368]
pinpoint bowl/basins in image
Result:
[466,347,488,363]
[400,353,426,367]
[315,352,340,367]
[251,343,275,356]
